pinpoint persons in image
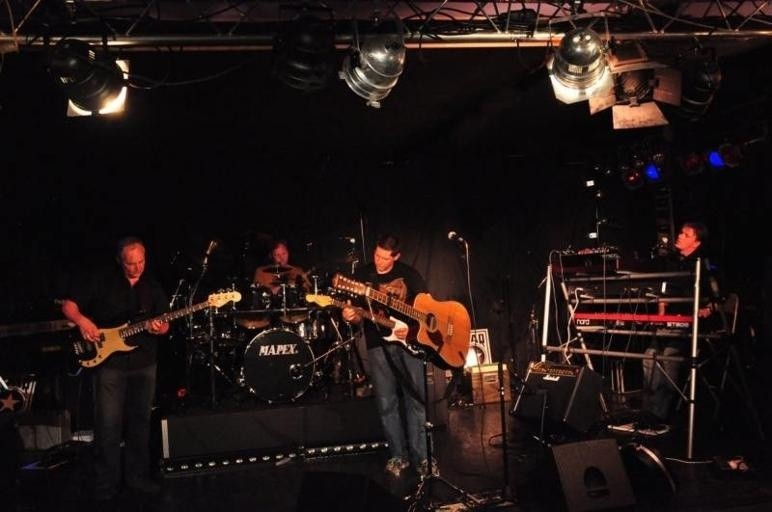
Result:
[59,234,174,506]
[636,214,715,420]
[341,236,441,481]
[249,237,312,301]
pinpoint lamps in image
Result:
[547,31,717,131]
[337,20,413,112]
[271,16,337,92]
[39,34,131,118]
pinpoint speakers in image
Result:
[543,440,637,511]
[510,362,602,441]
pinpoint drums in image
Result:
[239,328,315,403]
[234,283,274,329]
[276,286,310,324]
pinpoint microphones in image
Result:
[650,236,669,252]
[447,231,466,246]
[205,235,221,255]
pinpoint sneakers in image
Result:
[416,455,440,480]
[385,455,410,479]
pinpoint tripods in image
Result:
[402,363,482,512]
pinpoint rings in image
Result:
[85,334,89,338]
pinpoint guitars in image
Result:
[65,288,242,368]
[332,273,471,367]
[306,293,427,356]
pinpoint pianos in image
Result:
[574,312,693,328]
[564,271,694,288]
